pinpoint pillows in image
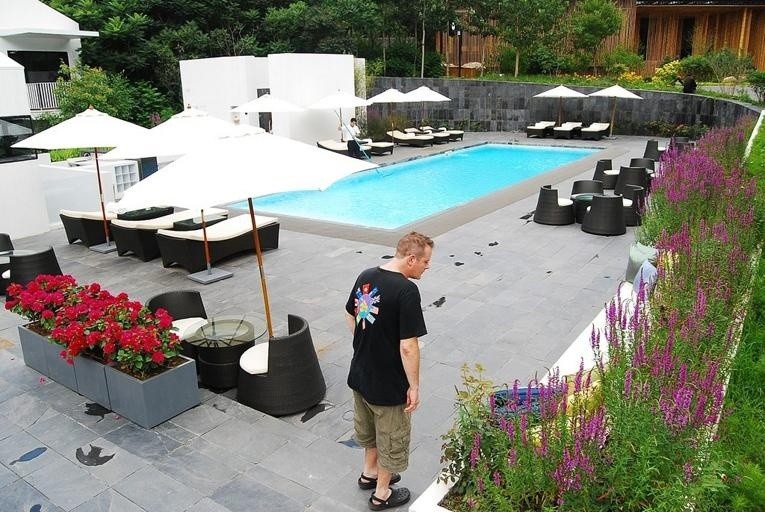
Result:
[626,241,659,298]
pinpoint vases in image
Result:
[18,320,198,430]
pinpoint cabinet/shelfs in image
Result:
[100,160,139,200]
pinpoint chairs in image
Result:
[59,207,280,274]
[534,137,695,235]
[0,233,63,301]
[145,290,326,417]
[317,126,463,159]
[527,121,610,141]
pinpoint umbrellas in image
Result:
[232,90,306,132]
[11,105,148,244]
[116,118,381,343]
[370,87,407,143]
[591,83,643,138]
[97,105,273,268]
[531,84,589,128]
[307,91,370,143]
[399,84,451,132]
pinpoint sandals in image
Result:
[369,487,410,511]
[358,472,401,490]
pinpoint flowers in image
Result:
[5,274,184,378]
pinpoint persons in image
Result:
[336,117,363,160]
[342,229,435,510]
[675,73,698,94]
